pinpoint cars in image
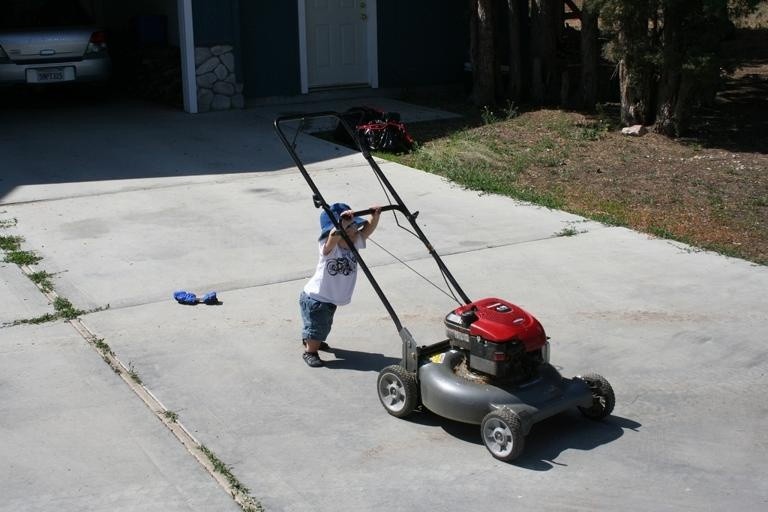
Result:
[0,0,116,101]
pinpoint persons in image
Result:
[296,202,382,369]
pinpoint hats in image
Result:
[318,202,368,241]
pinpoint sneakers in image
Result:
[303,338,330,350]
[303,351,322,368]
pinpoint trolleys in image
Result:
[260,98,621,466]
